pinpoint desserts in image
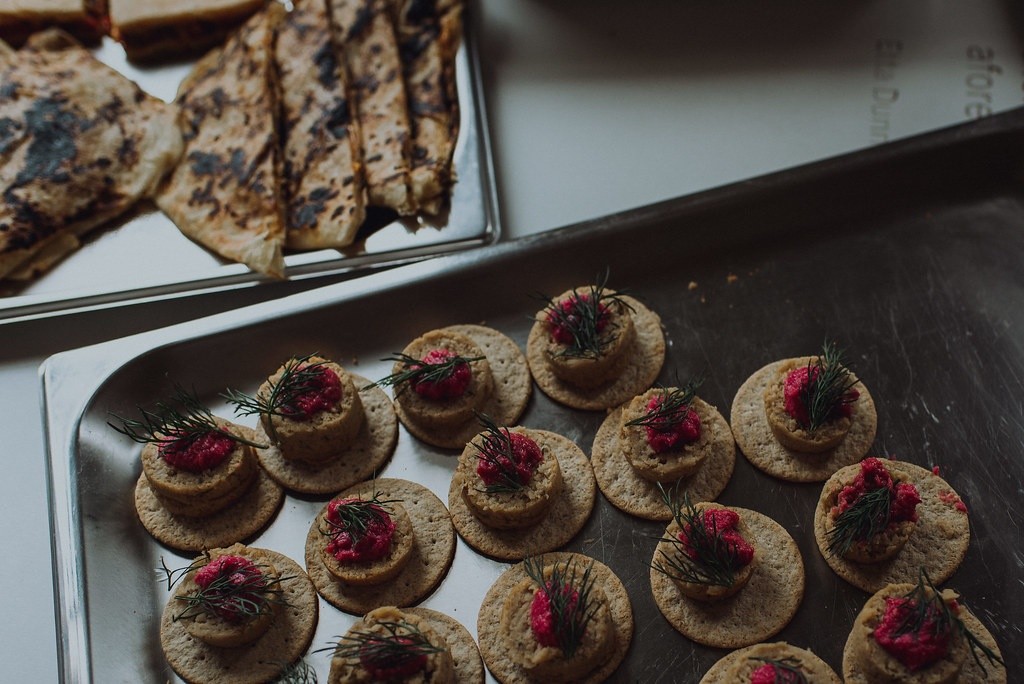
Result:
[101,273,1006,684]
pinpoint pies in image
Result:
[0,0,463,285]
